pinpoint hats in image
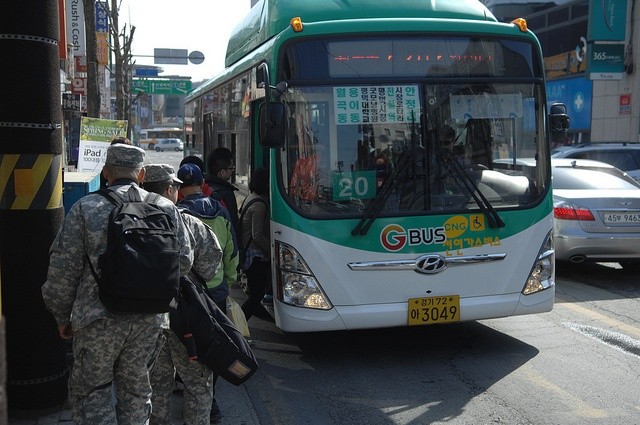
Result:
[106,142,146,169]
[177,165,204,186]
[140,165,184,185]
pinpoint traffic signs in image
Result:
[131,80,192,96]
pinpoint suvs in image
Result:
[548,140,640,187]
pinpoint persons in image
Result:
[239,167,272,316]
[433,122,473,197]
[42,144,195,425]
[168,153,228,211]
[175,163,239,314]
[140,164,222,425]
[206,146,240,230]
[111,136,132,146]
[288,135,332,204]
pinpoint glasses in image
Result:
[226,165,237,171]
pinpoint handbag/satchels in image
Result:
[176,273,256,387]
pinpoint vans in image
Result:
[154,139,184,153]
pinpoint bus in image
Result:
[139,127,187,151]
[181,0,572,336]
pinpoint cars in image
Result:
[493,157,640,273]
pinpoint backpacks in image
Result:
[97,186,178,316]
